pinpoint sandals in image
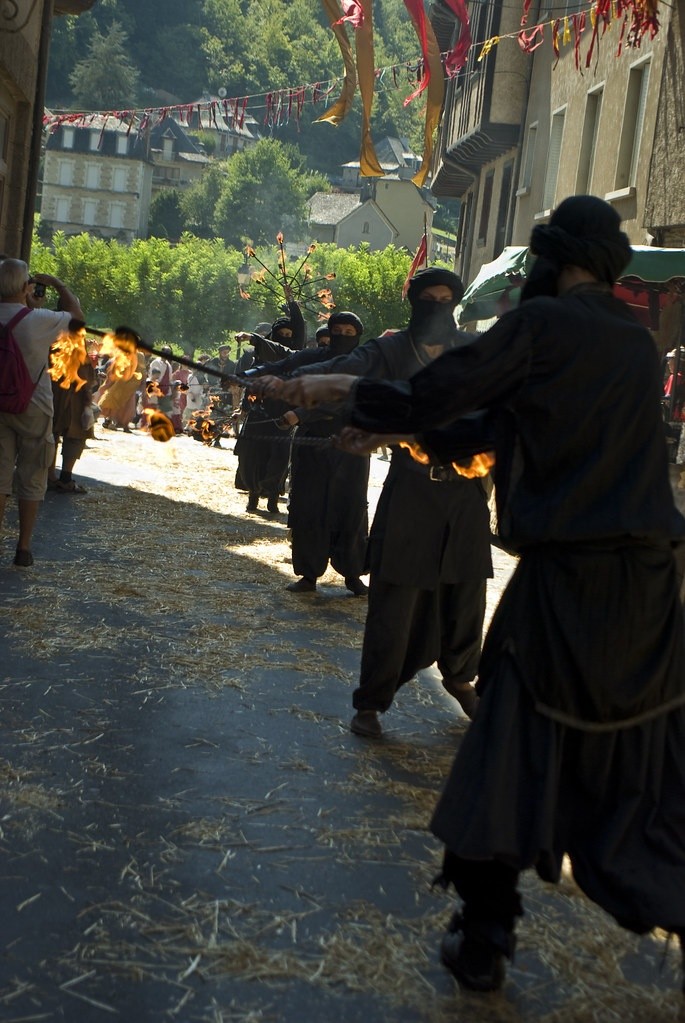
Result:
[45,478,59,490]
[56,477,85,494]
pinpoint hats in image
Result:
[313,324,330,342]
[407,267,465,307]
[271,318,296,332]
[527,194,631,277]
[326,312,364,334]
[254,322,272,334]
[217,345,231,352]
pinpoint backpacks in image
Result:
[0,307,46,415]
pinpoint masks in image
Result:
[272,332,293,348]
[527,260,553,296]
[330,333,356,352]
[415,299,451,329]
[318,343,327,347]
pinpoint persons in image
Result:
[0,271,394,493]
[274,196,685,992]
[662,347,685,463]
[0,257,84,569]
[249,265,497,739]
[231,284,308,515]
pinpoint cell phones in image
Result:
[34,283,46,297]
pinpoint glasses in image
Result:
[26,274,32,285]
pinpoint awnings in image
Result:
[453,244,685,328]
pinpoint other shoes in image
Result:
[284,576,315,592]
[266,495,279,514]
[343,578,370,596]
[14,548,32,567]
[442,676,478,721]
[245,494,259,511]
[438,915,506,992]
[352,706,379,735]
